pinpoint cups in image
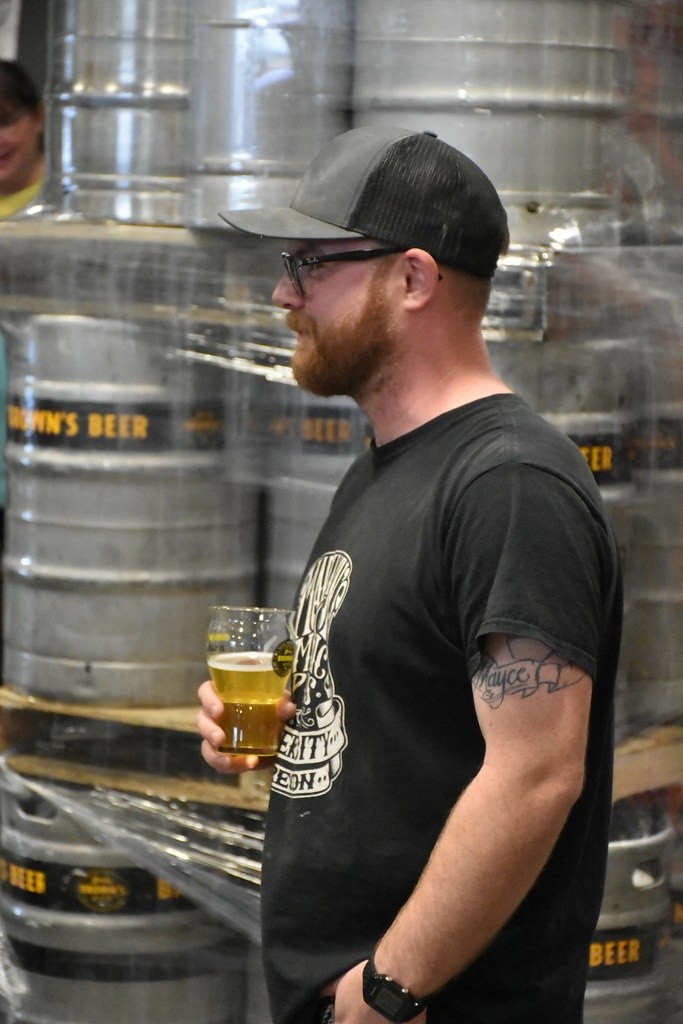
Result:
[208,606,293,754]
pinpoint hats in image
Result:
[217,126,503,279]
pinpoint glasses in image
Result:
[280,247,444,298]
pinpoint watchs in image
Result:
[362,953,426,1024]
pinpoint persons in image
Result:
[0,59,45,218]
[195,126,626,1024]
